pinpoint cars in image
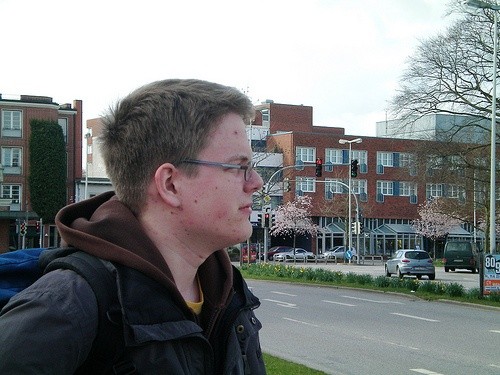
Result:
[319,245,356,263]
[385,249,435,281]
[273,248,315,263]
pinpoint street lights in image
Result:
[338,137,363,264]
[461,0,500,256]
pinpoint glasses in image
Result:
[172,159,257,181]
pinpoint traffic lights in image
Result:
[20,223,27,236]
[36,222,40,233]
[256,213,265,229]
[271,214,275,226]
[265,214,269,227]
[286,177,292,192]
[315,158,322,177]
[357,221,363,235]
[351,222,357,235]
[351,159,358,178]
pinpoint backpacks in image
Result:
[0,247,243,375]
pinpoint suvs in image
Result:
[258,246,293,261]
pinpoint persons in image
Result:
[0,84,266,375]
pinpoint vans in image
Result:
[242,245,257,263]
[443,240,484,273]
